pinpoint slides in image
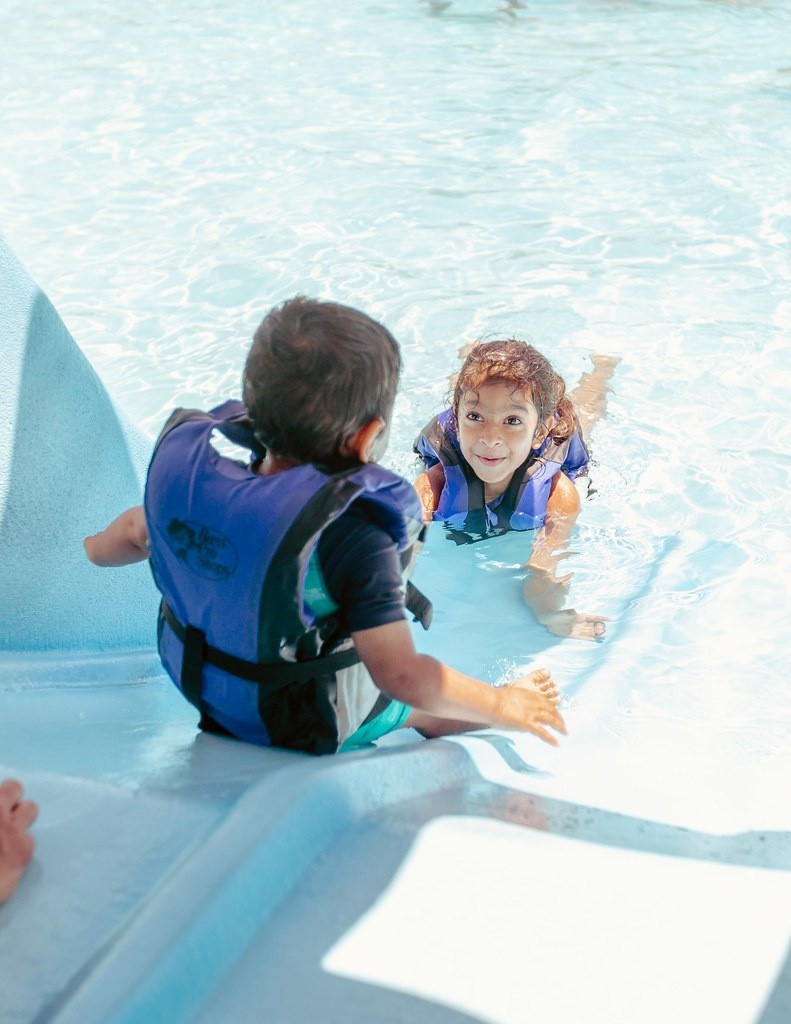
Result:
[0,518,791,1022]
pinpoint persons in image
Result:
[83,303,568,755]
[409,338,581,521]
